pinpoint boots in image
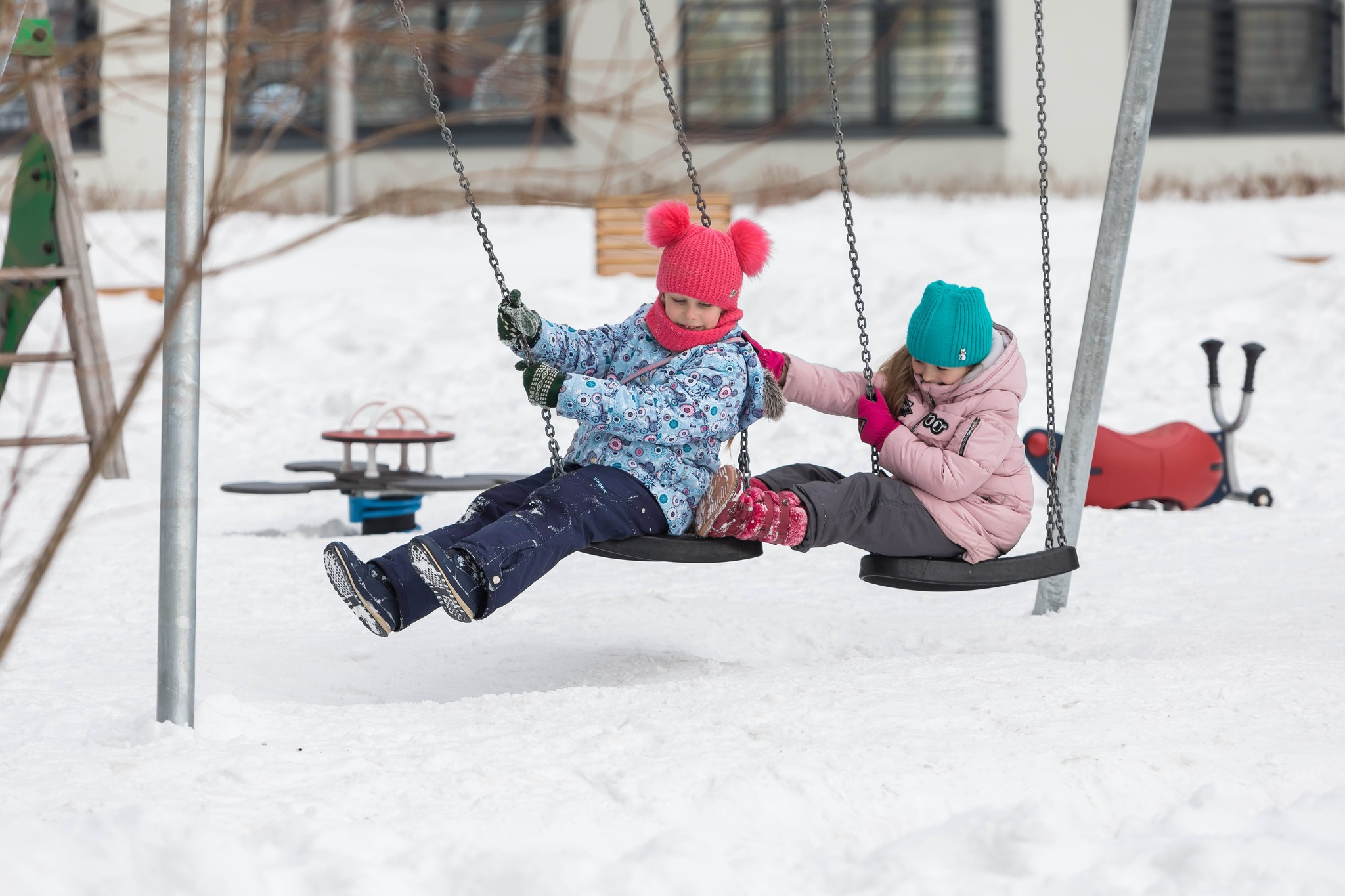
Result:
[743,476,768,492]
[693,465,808,547]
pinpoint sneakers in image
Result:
[408,535,486,623]
[324,541,398,637]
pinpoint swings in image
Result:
[819,1,1081,592]
[393,1,762,563]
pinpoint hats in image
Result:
[906,280,993,367]
[641,199,773,311]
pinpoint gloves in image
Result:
[741,329,785,384]
[857,385,902,453]
[515,360,566,408]
[497,289,542,351]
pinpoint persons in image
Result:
[694,280,1035,564]
[323,201,787,638]
